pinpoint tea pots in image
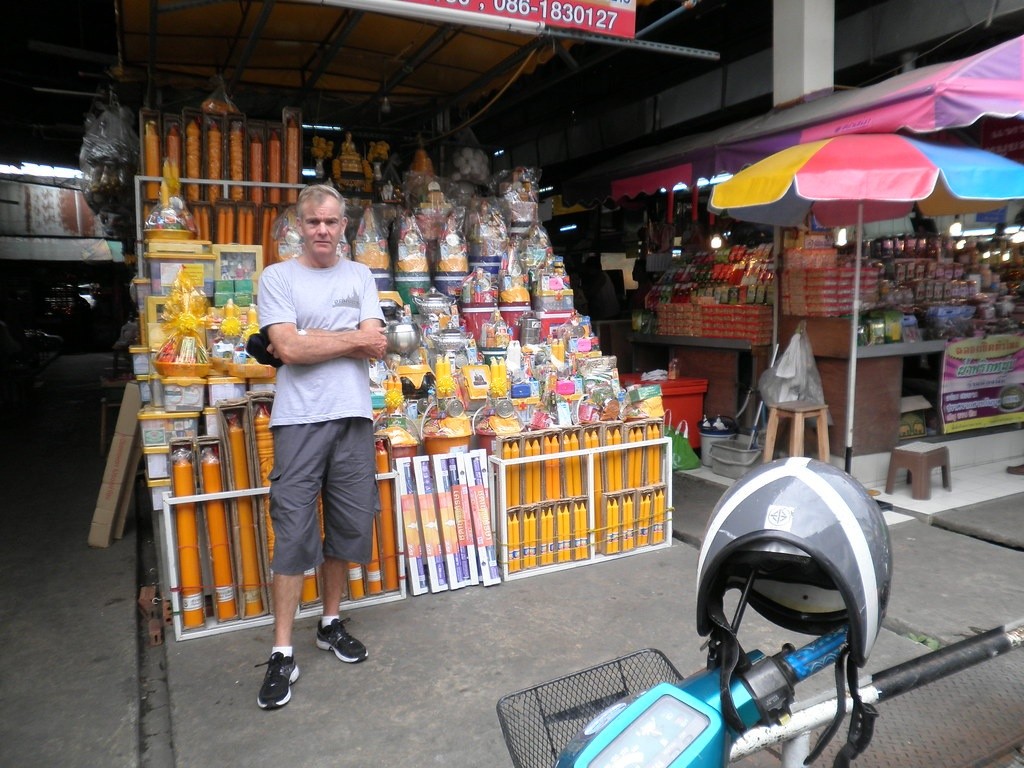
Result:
[430,322,467,354]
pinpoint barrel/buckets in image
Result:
[464,299,498,346]
[395,274,429,316]
[370,269,390,290]
[500,302,531,341]
[699,418,737,467]
[472,405,525,471]
[469,256,501,288]
[437,273,469,304]
[420,402,473,474]
[387,446,416,475]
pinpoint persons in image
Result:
[256,184,388,710]
[112,310,139,380]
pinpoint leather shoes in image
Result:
[1007,464,1024,475]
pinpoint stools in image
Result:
[885,441,952,501]
[762,400,830,464]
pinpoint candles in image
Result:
[169,341,667,634]
[141,108,302,332]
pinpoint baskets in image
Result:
[497,647,685,768]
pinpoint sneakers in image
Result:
[255,651,300,711]
[316,616,369,664]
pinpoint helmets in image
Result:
[694,456,894,668]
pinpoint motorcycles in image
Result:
[495,456,889,768]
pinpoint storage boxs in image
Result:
[128,240,276,484]
[619,373,708,459]
[898,395,933,441]
[530,288,575,347]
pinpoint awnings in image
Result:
[560,31,1024,217]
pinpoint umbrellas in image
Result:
[709,134,1024,473]
